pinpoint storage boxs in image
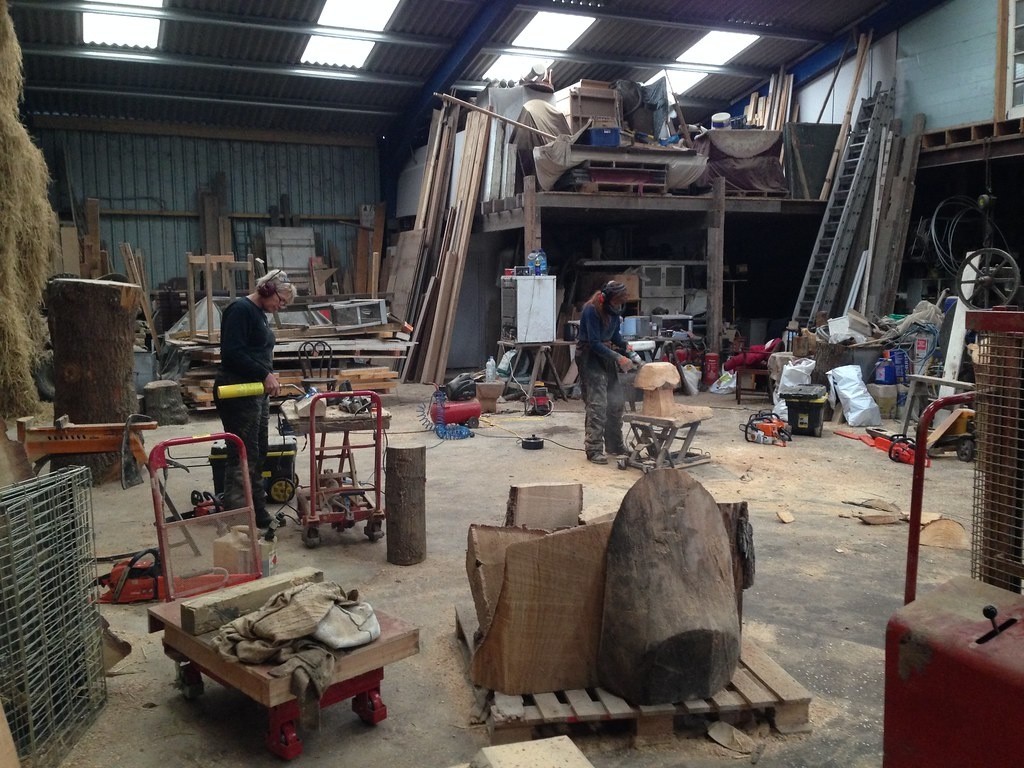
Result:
[896,384,919,419]
[785,392,829,438]
[827,307,872,337]
[867,383,897,419]
[884,349,912,385]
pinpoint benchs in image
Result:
[309,298,388,333]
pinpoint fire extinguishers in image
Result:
[698,349,718,385]
[663,341,692,365]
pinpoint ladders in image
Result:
[792,77,897,328]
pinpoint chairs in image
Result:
[724,338,785,405]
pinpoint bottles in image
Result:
[486,356,496,383]
[528,249,548,276]
[218,382,264,398]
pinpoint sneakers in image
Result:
[609,446,631,455]
[587,452,608,463]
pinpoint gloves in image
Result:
[625,344,634,354]
[618,356,633,371]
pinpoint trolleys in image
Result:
[146,433,424,760]
[291,389,390,550]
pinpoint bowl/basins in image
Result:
[505,269,514,276]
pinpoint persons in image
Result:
[575,279,644,464]
[212,268,298,528]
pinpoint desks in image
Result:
[186,251,254,344]
[497,340,577,410]
[616,405,714,473]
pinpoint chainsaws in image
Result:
[875,433,930,468]
[739,408,793,447]
[88,548,162,604]
[153,489,224,527]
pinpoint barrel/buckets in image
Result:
[875,359,896,384]
[711,112,732,129]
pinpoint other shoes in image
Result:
[256,508,273,527]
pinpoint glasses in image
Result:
[275,291,288,307]
